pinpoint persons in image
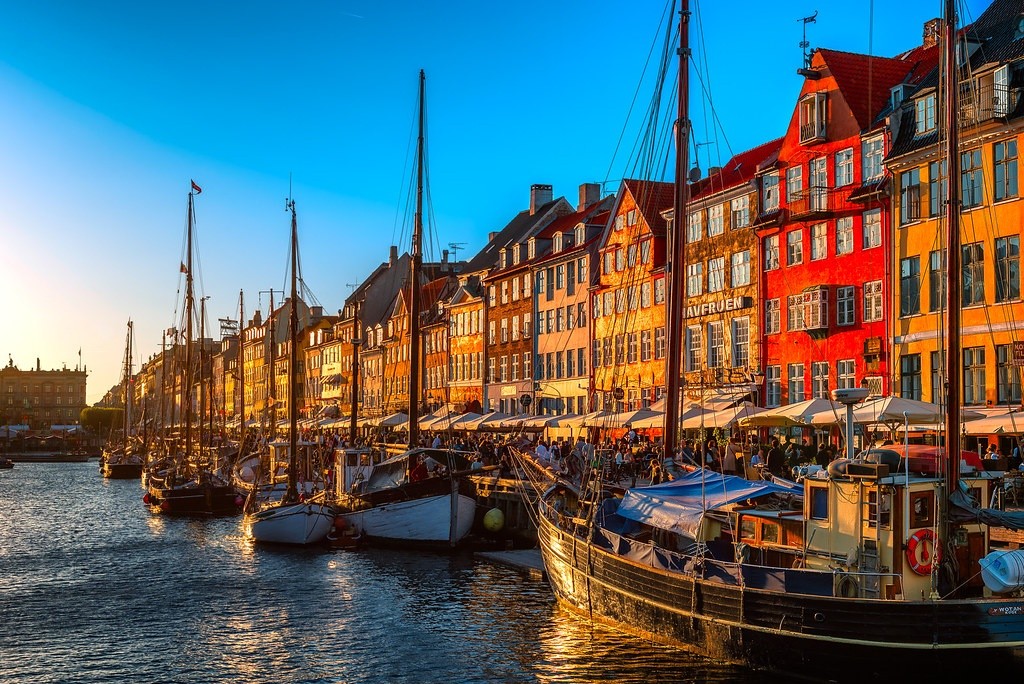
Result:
[150,426,836,489]
[984,439,1024,459]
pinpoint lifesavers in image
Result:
[906,528,943,577]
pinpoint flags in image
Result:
[192,182,201,191]
[167,328,176,336]
[180,261,188,274]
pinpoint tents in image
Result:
[0,428,90,447]
[163,395,1024,452]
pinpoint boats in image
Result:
[0,457,15,468]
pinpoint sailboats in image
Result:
[507,0,1023,683]
[98,68,504,552]
